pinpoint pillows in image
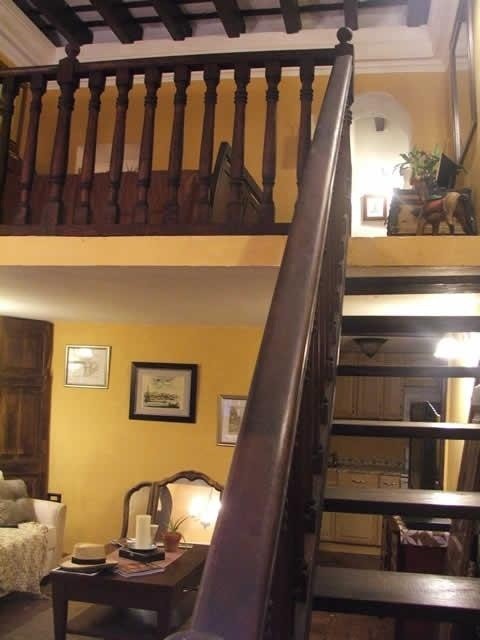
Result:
[1,478,36,526]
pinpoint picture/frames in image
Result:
[128,361,198,425]
[364,194,387,222]
[215,393,249,448]
[63,343,112,389]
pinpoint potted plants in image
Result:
[396,144,441,200]
[158,511,194,552]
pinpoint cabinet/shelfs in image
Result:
[316,469,336,544]
[335,470,402,546]
[336,351,446,421]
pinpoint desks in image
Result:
[385,189,473,236]
[378,513,450,639]
[46,545,211,639]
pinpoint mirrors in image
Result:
[447,0,477,165]
[119,468,223,546]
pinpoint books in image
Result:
[113,556,166,578]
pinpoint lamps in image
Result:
[353,338,388,357]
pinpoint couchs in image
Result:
[0,467,67,601]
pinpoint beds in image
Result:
[1,138,265,222]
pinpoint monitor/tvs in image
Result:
[408,400,452,491]
